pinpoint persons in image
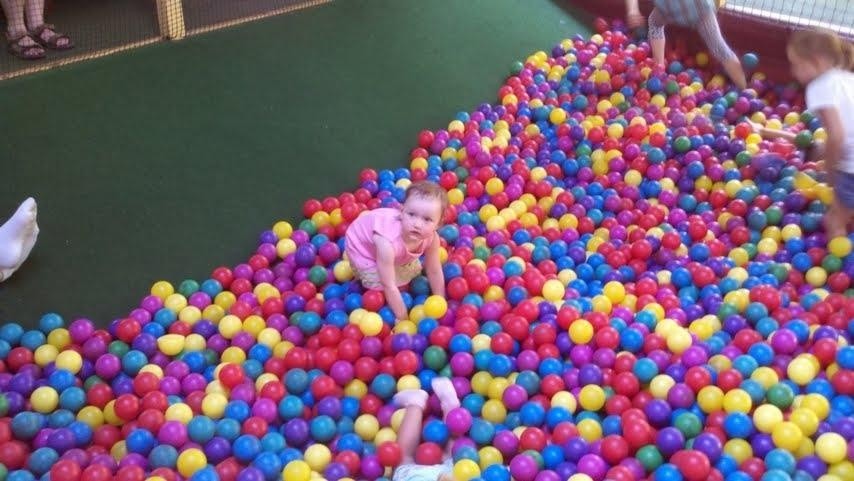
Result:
[1,0,77,59]
[621,0,749,90]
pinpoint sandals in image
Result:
[27,19,73,51]
[4,30,47,59]
